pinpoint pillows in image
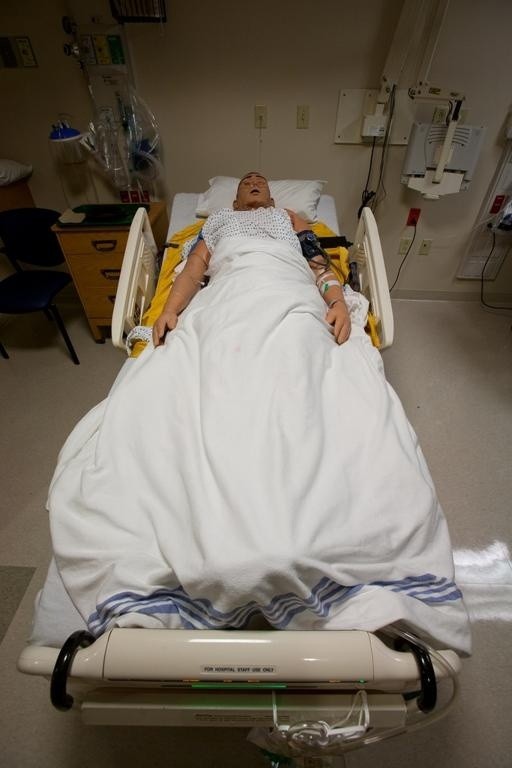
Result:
[0,158,34,187]
[195,173,327,223]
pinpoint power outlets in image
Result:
[482,218,497,234]
[407,208,421,226]
[255,105,267,128]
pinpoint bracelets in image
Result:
[327,297,345,308]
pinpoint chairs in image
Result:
[0,207,82,364]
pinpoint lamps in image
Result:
[48,119,82,143]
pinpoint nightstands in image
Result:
[51,199,170,343]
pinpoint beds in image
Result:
[17,191,475,730]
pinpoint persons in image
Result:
[152,170,351,348]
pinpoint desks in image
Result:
[0,178,36,212]
